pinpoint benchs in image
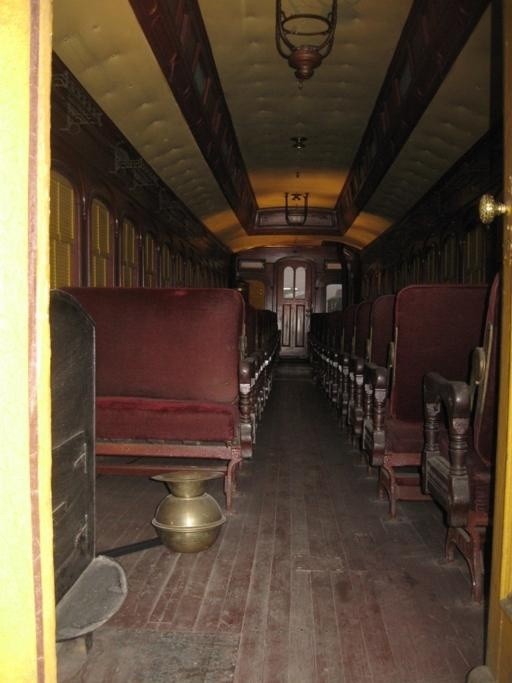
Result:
[63,280,282,509]
[308,272,504,606]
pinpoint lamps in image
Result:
[271,0,338,177]
[281,191,310,254]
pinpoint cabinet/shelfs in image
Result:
[52,289,100,606]
